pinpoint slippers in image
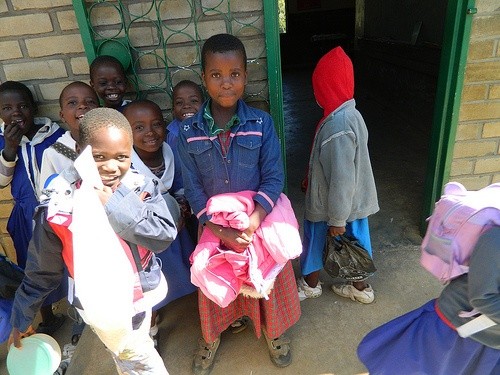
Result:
[36,313,65,336]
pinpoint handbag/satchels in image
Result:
[322,228,377,280]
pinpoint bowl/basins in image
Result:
[6,332,61,375]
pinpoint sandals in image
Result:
[230,316,249,334]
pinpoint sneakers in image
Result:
[192,332,221,375]
[295,276,322,302]
[261,327,291,367]
[331,282,375,304]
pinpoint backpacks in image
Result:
[420,182,500,286]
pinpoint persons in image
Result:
[6,107,182,375]
[357,226,500,375]
[176,33,302,375]
[294,46,380,304]
[0,54,248,375]
